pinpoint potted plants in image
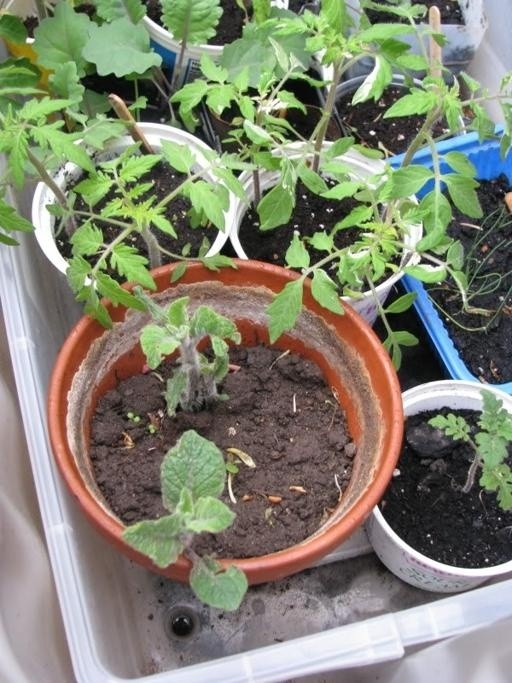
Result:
[366,379,512,594]
[47,255,404,588]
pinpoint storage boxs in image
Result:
[0,42,512,683]
